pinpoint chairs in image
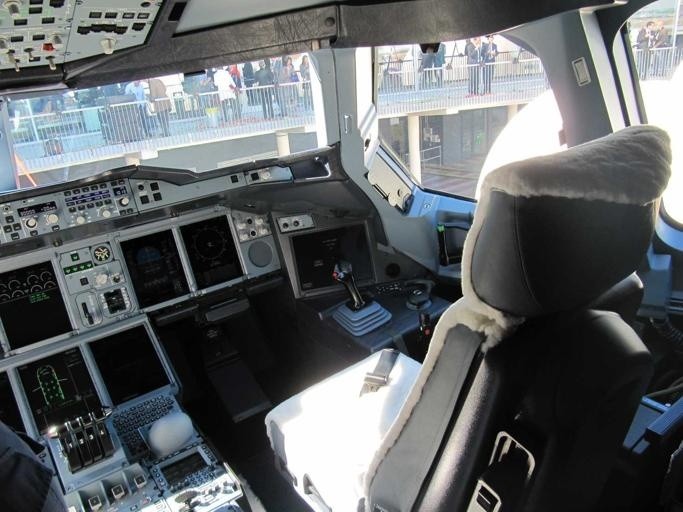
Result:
[265,126,673,512]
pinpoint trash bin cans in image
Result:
[206,108,219,128]
